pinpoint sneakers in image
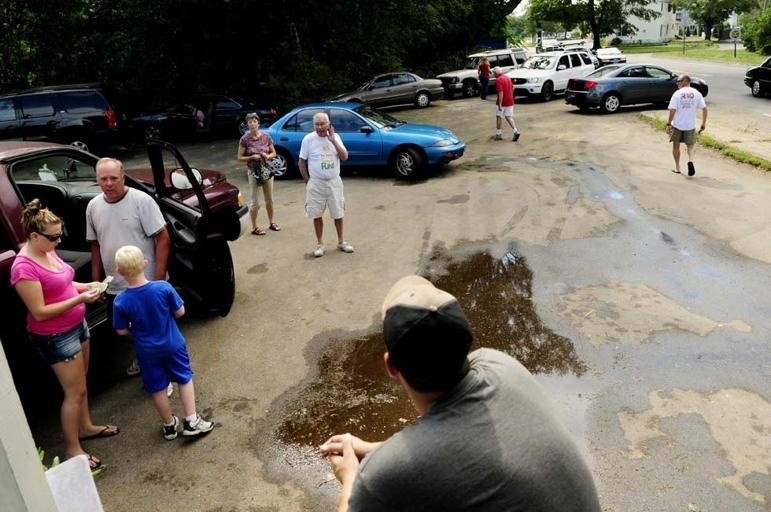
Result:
[313,244,326,257]
[168,383,173,397]
[182,413,215,436]
[496,133,501,140]
[511,130,520,143]
[126,360,140,375]
[162,414,180,440]
[337,241,354,253]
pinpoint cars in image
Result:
[592,47,626,67]
[241,102,467,181]
[321,71,445,109]
[564,63,708,114]
[115,93,280,156]
[743,58,771,100]
[535,34,590,54]
[503,41,600,101]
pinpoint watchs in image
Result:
[666,123,671,126]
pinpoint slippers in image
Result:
[687,161,695,176]
[79,424,119,440]
[87,454,102,470]
[672,169,681,173]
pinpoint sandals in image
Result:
[251,226,266,235]
[269,222,281,231]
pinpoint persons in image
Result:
[493,66,521,141]
[87,158,172,400]
[238,112,280,234]
[478,56,493,102]
[11,201,119,471]
[111,245,216,440]
[188,105,204,126]
[298,113,353,257]
[321,275,604,512]
[665,74,707,176]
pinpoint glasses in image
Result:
[676,78,684,83]
[33,230,63,243]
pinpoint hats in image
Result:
[382,275,474,388]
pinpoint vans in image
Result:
[0,82,132,161]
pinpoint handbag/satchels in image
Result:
[251,155,276,186]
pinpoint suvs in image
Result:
[0,139,249,392]
[435,52,524,102]
